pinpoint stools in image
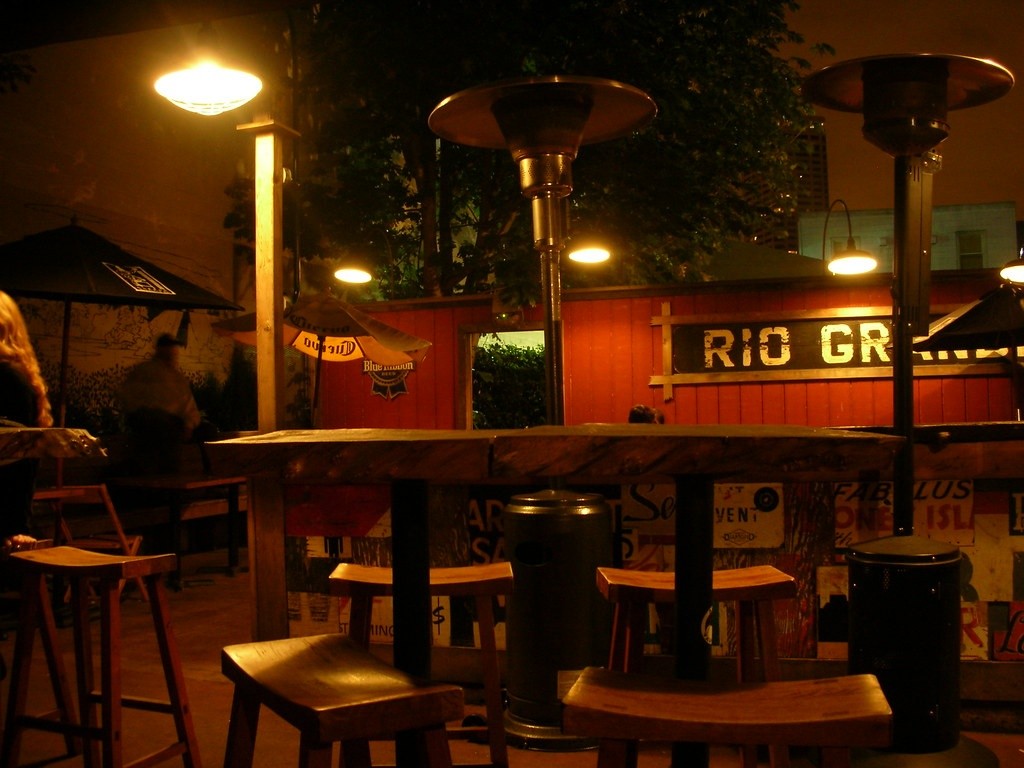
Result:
[0,544,203,768]
[220,632,467,768]
[321,559,518,768]
[560,667,894,768]
[594,563,800,768]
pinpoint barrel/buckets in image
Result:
[847,537,961,754]
[506,490,612,752]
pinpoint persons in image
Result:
[115,330,205,593]
[0,290,52,681]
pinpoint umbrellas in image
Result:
[912,281,1024,422]
[0,216,246,484]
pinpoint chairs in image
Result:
[52,484,150,602]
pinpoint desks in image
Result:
[204,423,913,768]
[107,471,247,593]
